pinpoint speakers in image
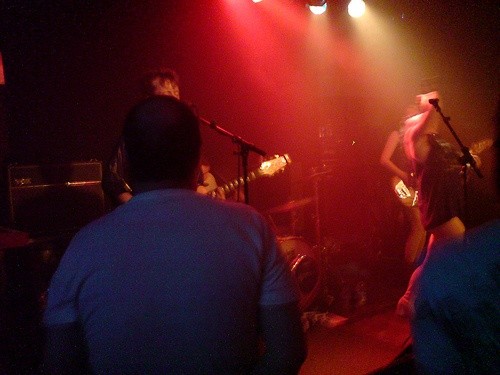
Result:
[7,162,104,235]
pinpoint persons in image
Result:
[411,94,500,374]
[380,99,469,261]
[102,60,210,204]
[39,93,309,375]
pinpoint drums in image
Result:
[277,236,321,311]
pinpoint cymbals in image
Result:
[271,197,313,212]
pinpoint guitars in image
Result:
[204,154,291,200]
[390,139,493,207]
